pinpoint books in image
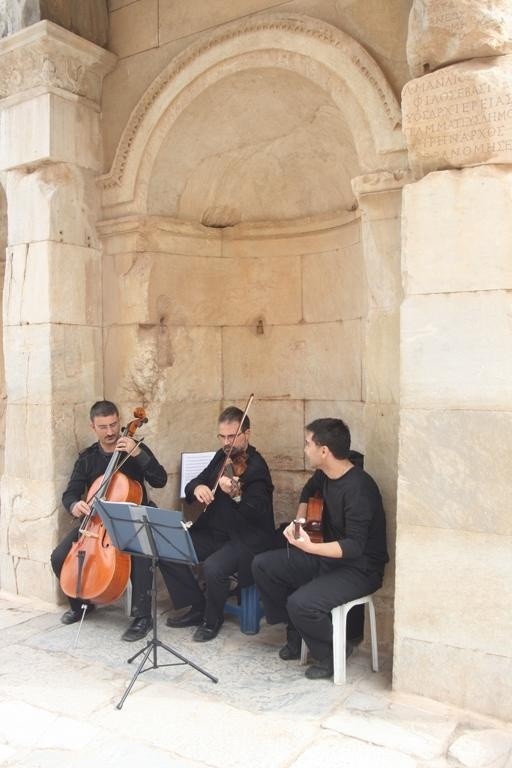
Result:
[93,497,200,566]
[179,451,217,500]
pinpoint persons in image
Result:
[49,399,168,643]
[157,405,277,643]
[248,416,390,682]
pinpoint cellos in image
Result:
[60,407,149,606]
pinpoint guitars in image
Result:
[292,491,325,543]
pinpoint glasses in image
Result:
[217,431,244,441]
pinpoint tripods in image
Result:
[113,559,220,711]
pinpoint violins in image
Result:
[221,449,248,499]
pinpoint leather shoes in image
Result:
[278,639,302,661]
[59,599,95,625]
[122,614,154,642]
[192,614,225,642]
[166,606,205,628]
[304,638,353,680]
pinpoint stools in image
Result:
[202,581,269,636]
[300,595,380,686]
